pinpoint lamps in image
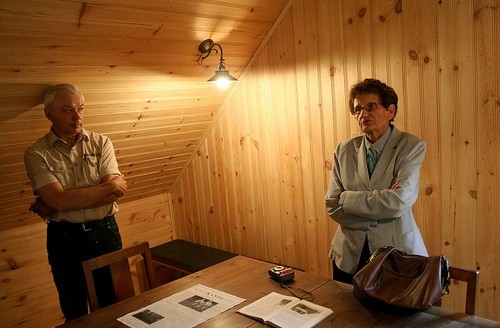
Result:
[199,39,238,89]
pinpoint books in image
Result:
[235,291,335,328]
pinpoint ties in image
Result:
[366,145,379,177]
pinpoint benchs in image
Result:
[152,239,306,281]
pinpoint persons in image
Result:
[325,78,429,284]
[193,298,212,310]
[24,83,127,323]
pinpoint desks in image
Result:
[55,255,333,328]
[250,281,500,328]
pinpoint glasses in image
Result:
[353,101,384,113]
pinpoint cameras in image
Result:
[269,265,295,282]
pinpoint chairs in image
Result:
[432,266,480,316]
[83,242,160,313]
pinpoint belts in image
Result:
[48,215,114,232]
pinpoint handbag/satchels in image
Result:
[352,245,452,311]
